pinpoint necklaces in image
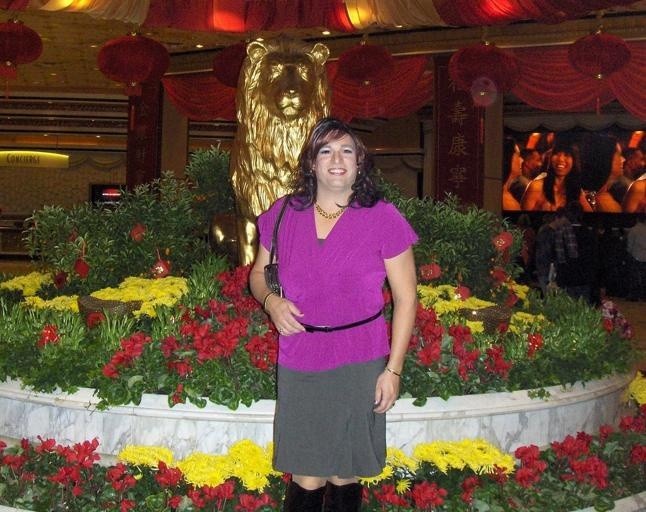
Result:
[314,202,348,219]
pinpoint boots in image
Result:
[282,478,364,512]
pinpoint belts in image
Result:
[300,310,382,333]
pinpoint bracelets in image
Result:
[262,291,279,311]
[385,366,401,377]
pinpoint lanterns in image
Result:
[96,34,171,85]
[449,44,523,96]
[213,42,251,87]
[0,23,44,63]
[568,34,631,79]
[338,42,390,83]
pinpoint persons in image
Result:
[503,130,646,211]
[249,118,419,512]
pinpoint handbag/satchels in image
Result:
[264,264,280,294]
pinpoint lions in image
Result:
[210,35,333,270]
